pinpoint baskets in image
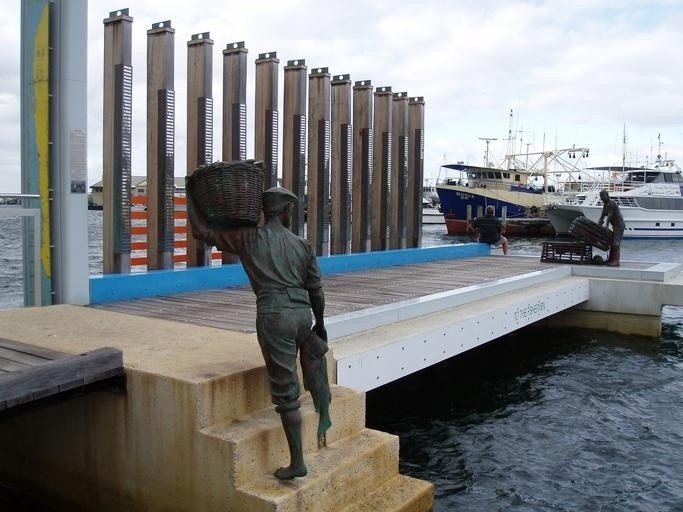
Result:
[184,159,269,228]
[569,216,614,251]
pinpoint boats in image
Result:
[422,136,508,225]
[542,131,682,242]
[434,106,656,239]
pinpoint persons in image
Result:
[469,205,508,256]
[598,190,625,266]
[185,160,332,480]
